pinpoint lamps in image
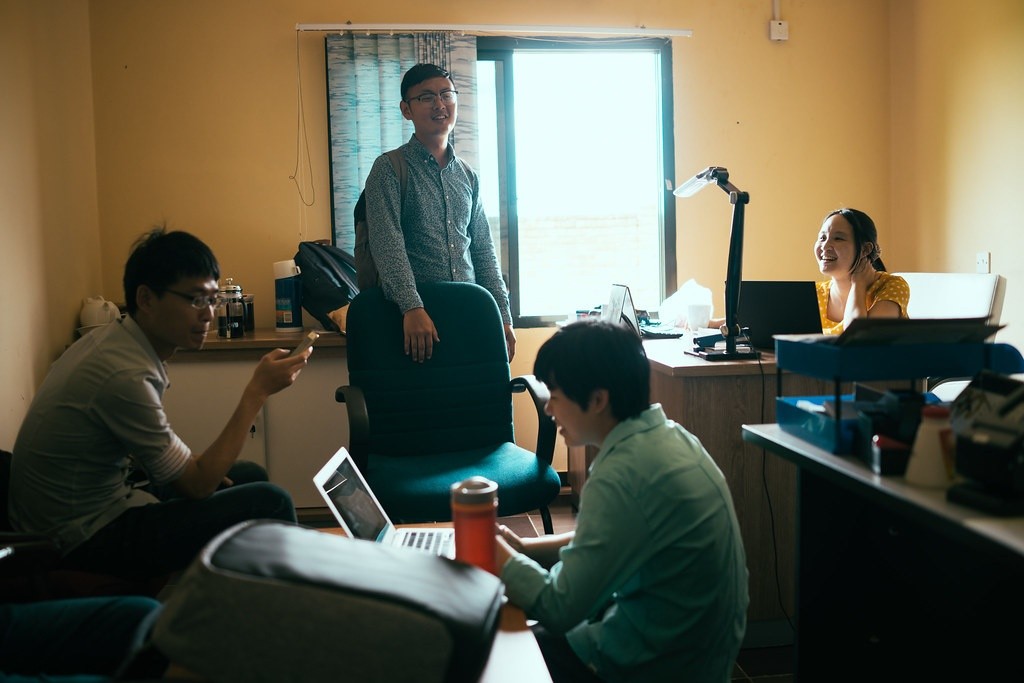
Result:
[674,166,762,360]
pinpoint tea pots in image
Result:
[80,296,121,326]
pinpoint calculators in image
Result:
[641,326,683,339]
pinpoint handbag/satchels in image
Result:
[152,520,508,683]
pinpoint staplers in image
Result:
[693,328,751,351]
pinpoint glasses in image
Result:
[406,90,459,108]
[153,288,227,310]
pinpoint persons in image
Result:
[493,321,749,683]
[10,228,312,566]
[364,62,518,364]
[710,209,910,335]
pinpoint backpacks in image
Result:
[293,241,359,332]
[354,150,408,293]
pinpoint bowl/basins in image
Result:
[76,323,108,337]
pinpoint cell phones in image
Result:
[290,331,318,357]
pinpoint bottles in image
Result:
[215,278,243,339]
[273,259,304,333]
[450,476,498,575]
[243,293,255,332]
[903,406,957,484]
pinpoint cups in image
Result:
[688,304,712,331]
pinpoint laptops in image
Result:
[724,280,824,349]
[312,446,457,561]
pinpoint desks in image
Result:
[742,424,1024,683]
[174,328,348,357]
[556,321,927,648]
[480,597,555,683]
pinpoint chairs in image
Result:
[893,271,1006,343]
[336,283,556,534]
